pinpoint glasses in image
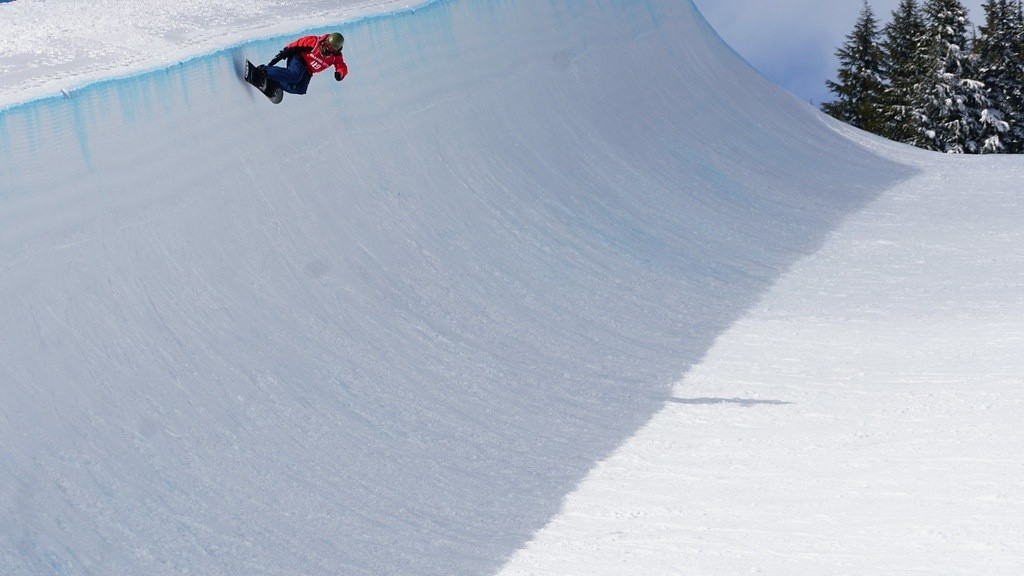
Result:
[324,37,336,54]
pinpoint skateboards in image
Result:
[243,60,283,105]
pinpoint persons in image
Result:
[257,32,348,94]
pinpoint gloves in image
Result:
[275,51,287,60]
[335,72,342,81]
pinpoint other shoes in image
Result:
[250,64,266,86]
[265,78,278,97]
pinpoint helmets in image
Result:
[324,33,344,52]
